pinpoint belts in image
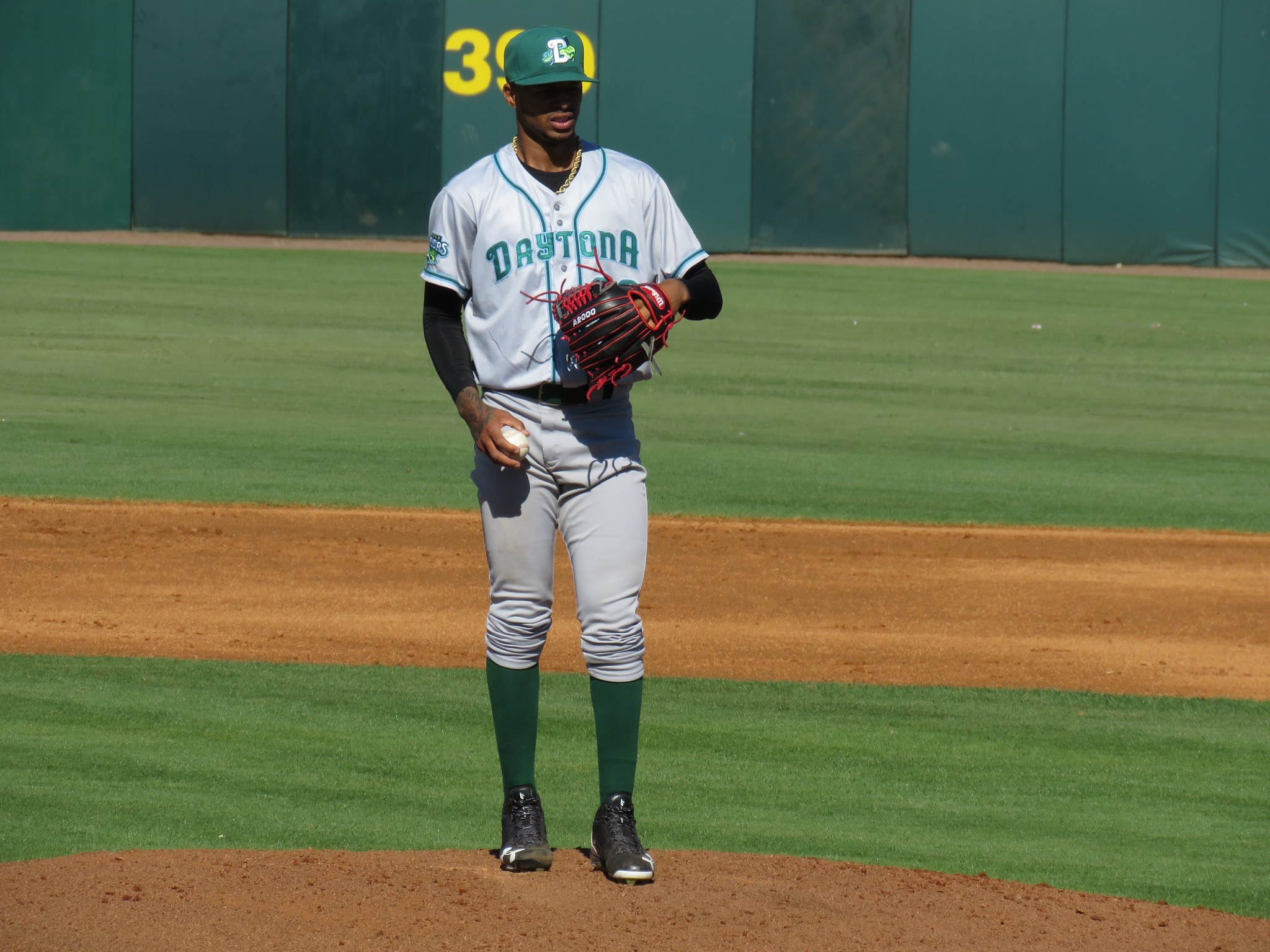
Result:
[509,382,615,406]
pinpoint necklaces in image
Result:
[512,135,583,195]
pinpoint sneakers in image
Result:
[590,793,656,879]
[499,785,554,869]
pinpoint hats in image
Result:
[503,26,600,85]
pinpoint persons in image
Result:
[420,25,724,881]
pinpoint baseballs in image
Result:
[495,425,529,462]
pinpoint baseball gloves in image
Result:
[552,284,675,388]
